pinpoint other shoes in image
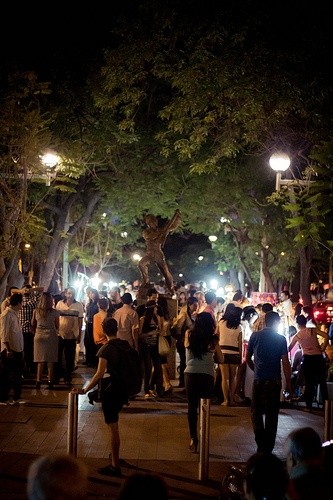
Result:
[35,381,42,390]
[47,379,54,390]
[99,465,123,478]
[65,381,74,389]
[0,398,31,407]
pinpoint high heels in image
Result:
[188,438,198,453]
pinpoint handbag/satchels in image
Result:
[157,334,170,358]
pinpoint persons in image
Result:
[0,279,333,409]
[120,473,167,500]
[243,452,288,500]
[287,315,329,411]
[184,312,224,453]
[28,453,87,500]
[138,209,181,300]
[285,427,333,500]
[132,300,169,401]
[0,292,23,407]
[247,314,290,455]
[214,307,244,405]
[31,292,59,390]
[51,287,83,389]
[71,318,143,476]
